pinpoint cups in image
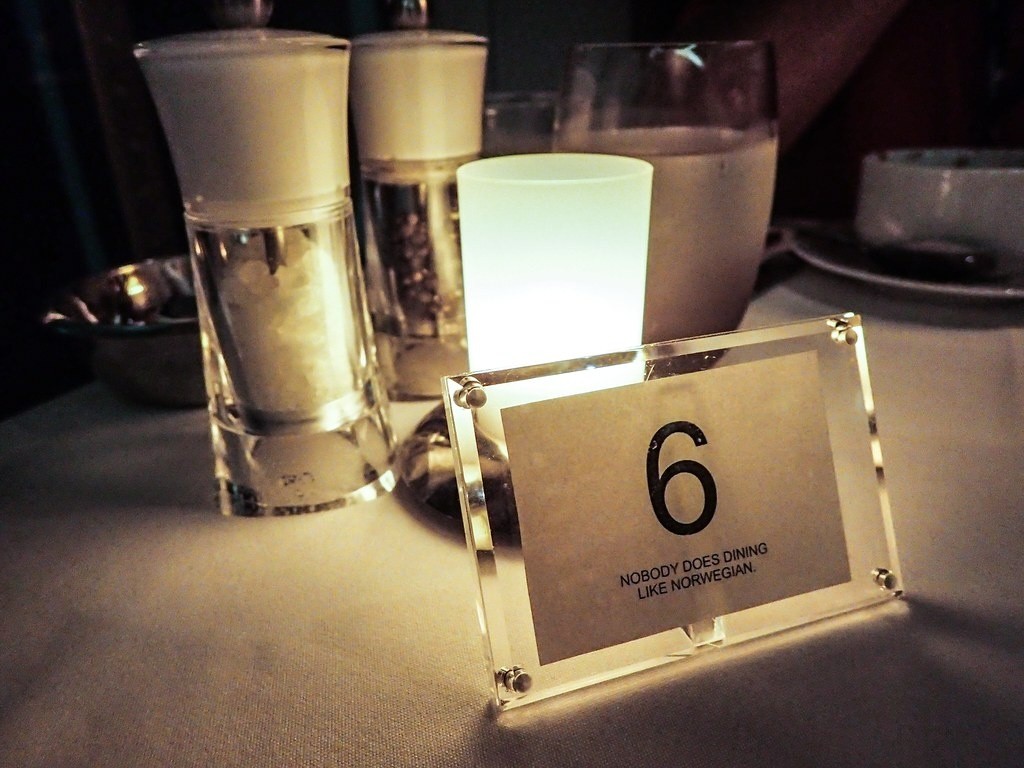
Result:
[134,0,402,517]
[347,0,490,404]
[852,145,1024,274]
[550,40,780,377]
[478,87,551,161]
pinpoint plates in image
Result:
[782,220,1024,303]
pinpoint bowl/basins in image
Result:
[43,253,205,409]
